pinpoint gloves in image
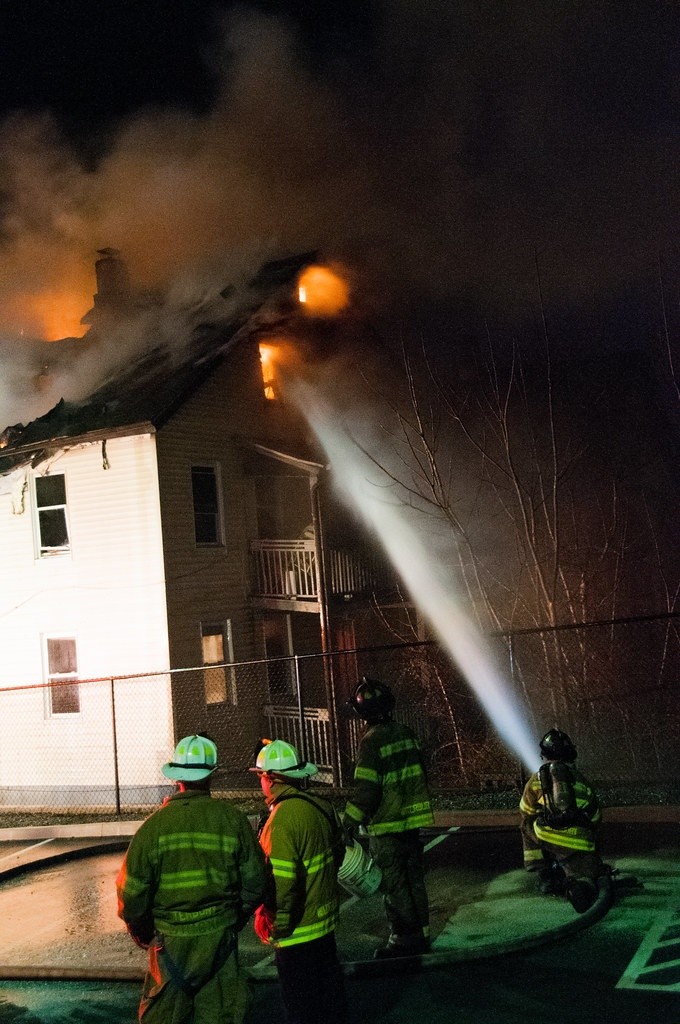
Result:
[342,814,360,847]
[127,920,155,949]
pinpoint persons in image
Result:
[340,675,436,959]
[114,730,270,1024]
[250,738,352,1024]
[517,728,611,896]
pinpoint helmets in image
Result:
[349,677,395,719]
[162,736,219,782]
[249,740,318,779]
[540,729,575,760]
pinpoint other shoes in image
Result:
[535,876,554,893]
[374,932,431,963]
[567,876,595,913]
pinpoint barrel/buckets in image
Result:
[335,837,383,898]
